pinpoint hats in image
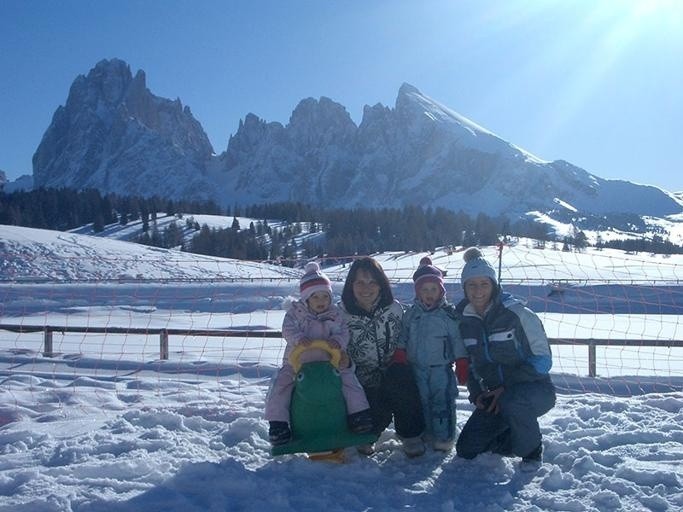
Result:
[461,249,499,288]
[300,262,332,308]
[413,257,445,301]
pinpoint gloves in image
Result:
[456,358,466,384]
[396,349,408,365]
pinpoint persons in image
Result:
[394,256,470,452]
[267,262,373,447]
[333,257,426,458]
[456,248,556,472]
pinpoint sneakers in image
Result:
[522,444,543,471]
[269,428,291,444]
[403,434,454,456]
[348,413,373,434]
